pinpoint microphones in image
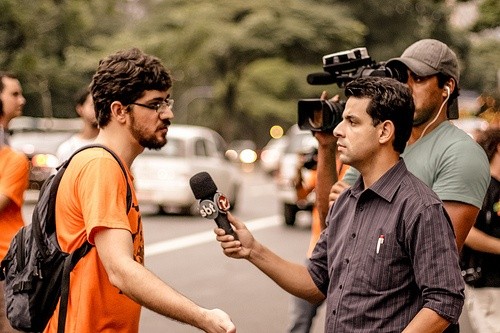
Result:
[190,172,238,241]
[307,72,352,85]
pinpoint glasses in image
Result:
[133,99,175,113]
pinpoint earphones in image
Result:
[444,84,449,91]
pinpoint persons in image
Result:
[213,39,500,333]
[0,71,31,333]
[42,49,236,333]
[48,85,104,176]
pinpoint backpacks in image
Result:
[0,144,132,333]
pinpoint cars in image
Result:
[278,116,337,225]
[131,125,242,215]
[261,133,286,174]
[9,116,93,189]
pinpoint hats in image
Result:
[385,38,460,85]
